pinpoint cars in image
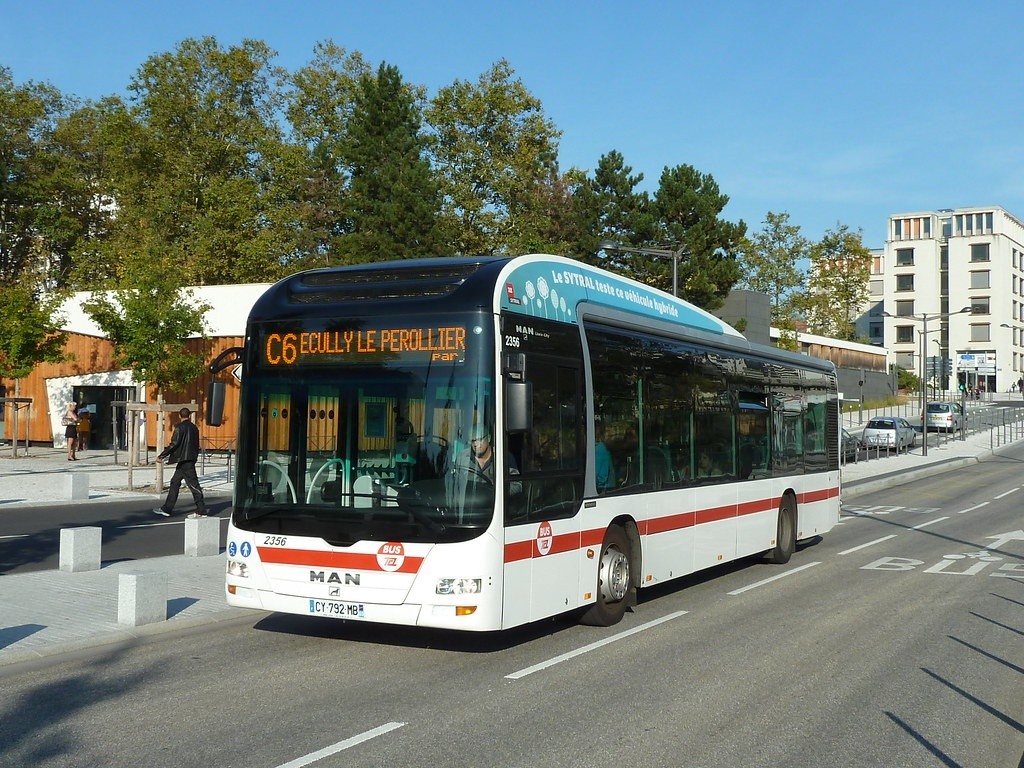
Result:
[861,416,918,452]
[840,427,862,464]
[920,401,969,434]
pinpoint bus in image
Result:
[204,255,842,634]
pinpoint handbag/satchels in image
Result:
[61,411,77,426]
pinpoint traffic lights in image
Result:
[958,371,967,392]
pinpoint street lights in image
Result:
[877,306,974,457]
[596,240,678,298]
[918,327,947,409]
[933,339,941,403]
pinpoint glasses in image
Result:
[472,434,488,442]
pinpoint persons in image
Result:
[959,383,980,400]
[65,402,90,461]
[1017,377,1024,393]
[1012,383,1017,393]
[455,426,523,497]
[153,408,208,517]
[540,415,816,493]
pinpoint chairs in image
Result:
[412,434,449,481]
[607,448,633,487]
[696,436,818,476]
[643,445,671,485]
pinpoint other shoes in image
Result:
[186,511,208,518]
[68,457,76,461]
[153,507,171,517]
